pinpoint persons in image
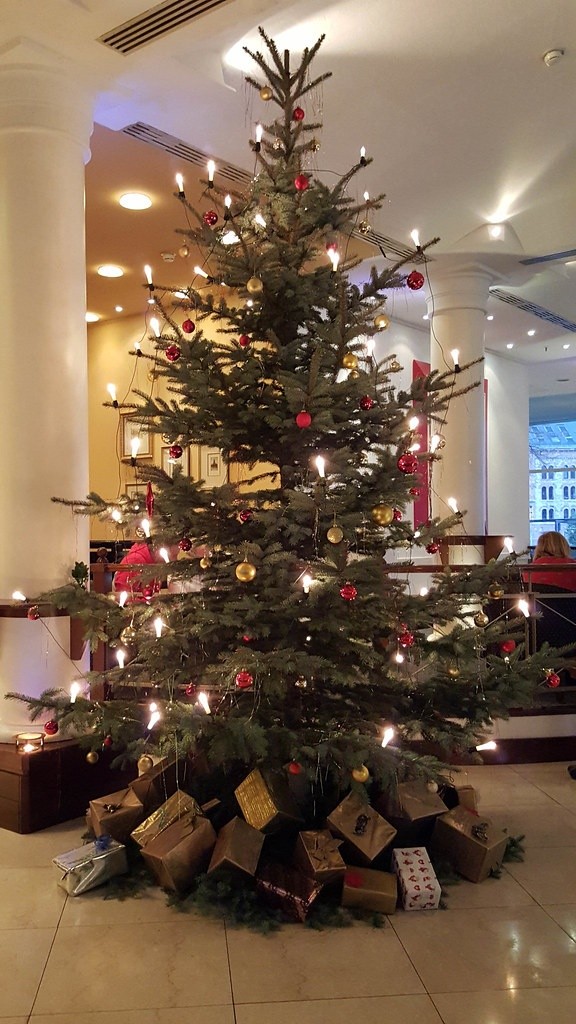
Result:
[111,531,181,606]
[521,531,576,592]
